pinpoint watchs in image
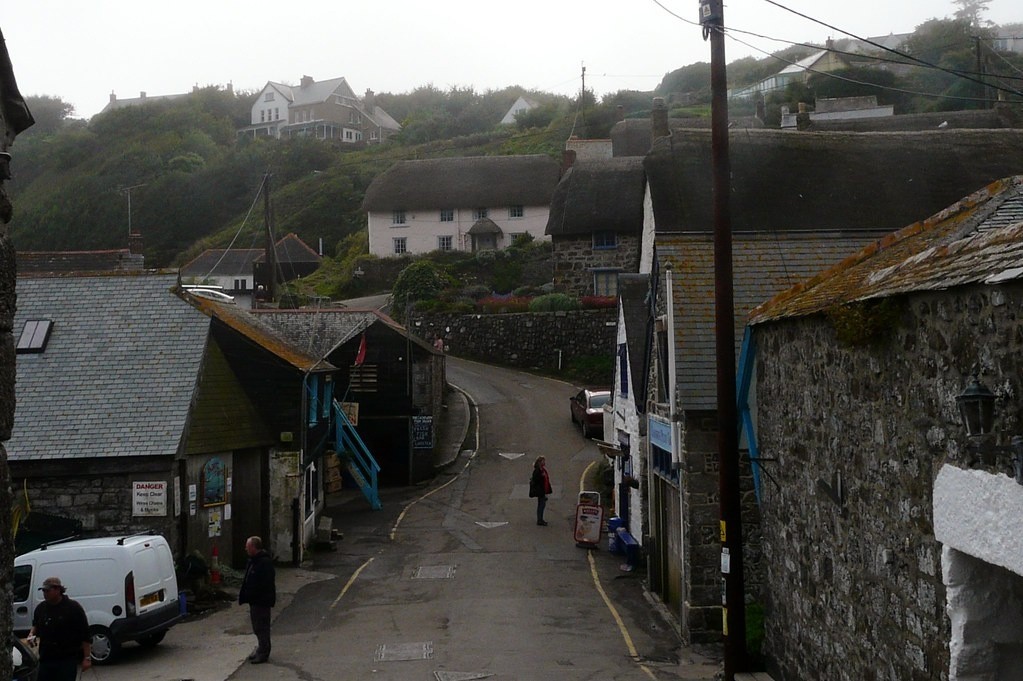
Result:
[83,655,91,661]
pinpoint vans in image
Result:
[11,536,183,665]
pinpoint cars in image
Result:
[188,290,236,307]
[569,387,612,438]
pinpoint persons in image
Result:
[255,283,267,309]
[528,456,554,526]
[240,535,277,665]
[24,577,94,681]
[433,332,444,353]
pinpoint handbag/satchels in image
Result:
[529,470,540,498]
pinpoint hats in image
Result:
[38,577,61,590]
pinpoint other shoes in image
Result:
[249,648,269,664]
[537,520,548,526]
